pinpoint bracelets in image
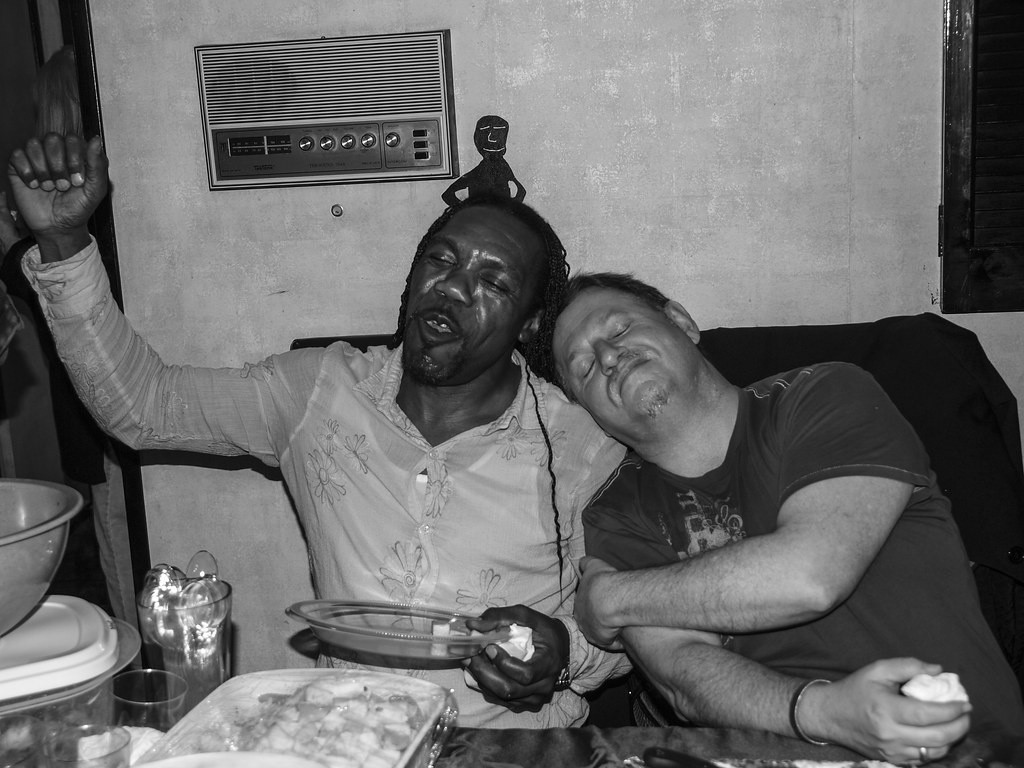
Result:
[790,678,831,746]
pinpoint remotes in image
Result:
[643,746,720,768]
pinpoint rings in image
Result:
[920,747,927,762]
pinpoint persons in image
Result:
[6,133,633,729]
[533,272,1024,768]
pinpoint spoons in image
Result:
[143,551,226,648]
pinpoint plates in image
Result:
[285,601,511,661]
[0,616,143,716]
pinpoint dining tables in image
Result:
[434,725,1024,768]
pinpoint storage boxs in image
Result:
[0,594,142,768]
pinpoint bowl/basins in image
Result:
[0,478,84,637]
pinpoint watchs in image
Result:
[556,656,570,690]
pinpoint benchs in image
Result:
[288,312,1024,710]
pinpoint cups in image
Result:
[108,670,187,732]
[136,579,234,727]
[0,715,46,768]
[47,725,132,768]
